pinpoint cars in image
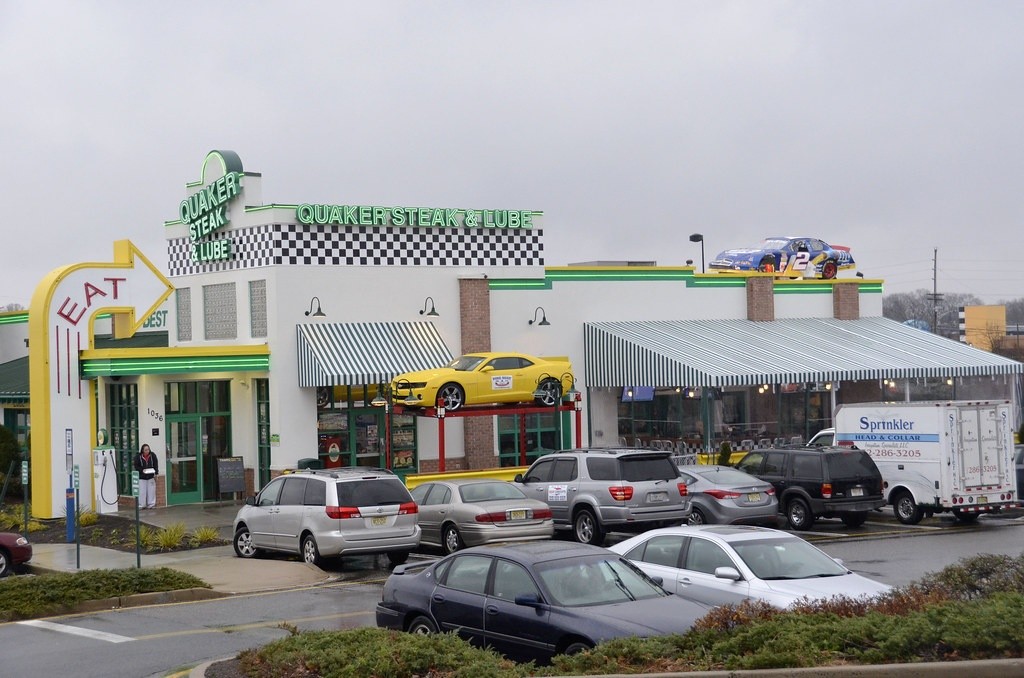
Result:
[374,540,733,671]
[576,524,904,616]
[1014,443,1024,502]
[0,531,33,577]
[677,462,780,529]
[405,476,554,556]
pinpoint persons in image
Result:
[134,444,159,511]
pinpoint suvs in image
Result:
[731,443,887,532]
[504,444,692,546]
[232,467,423,571]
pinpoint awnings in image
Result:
[295,321,455,387]
[584,314,1024,388]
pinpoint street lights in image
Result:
[688,232,706,274]
[371,378,419,471]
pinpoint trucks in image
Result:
[792,400,1024,526]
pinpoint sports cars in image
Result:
[385,351,576,413]
[709,236,856,282]
[316,383,392,410]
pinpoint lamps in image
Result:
[528,306,551,326]
[304,297,326,317]
[419,297,439,317]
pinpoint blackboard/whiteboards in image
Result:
[216,456,245,491]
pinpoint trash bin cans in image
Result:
[393,468,416,485]
[298,458,321,469]
